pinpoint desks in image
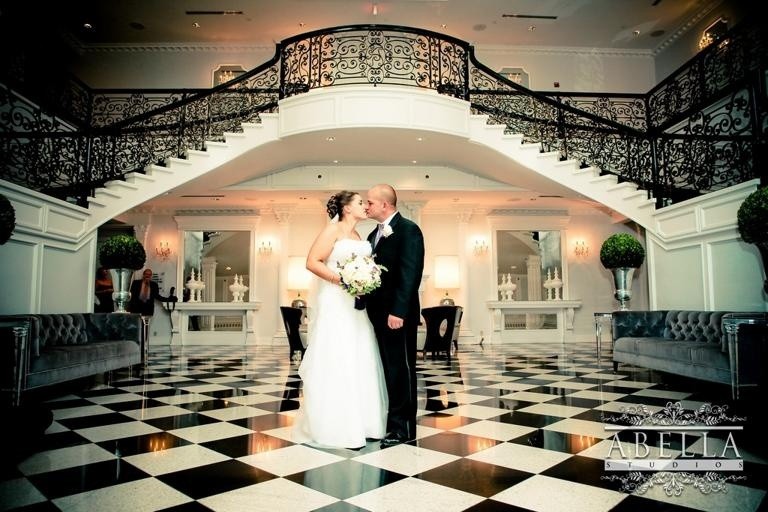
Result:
[594,312,614,368]
[130,311,150,368]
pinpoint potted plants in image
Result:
[737,188,766,299]
[96,235,145,318]
[600,230,648,312]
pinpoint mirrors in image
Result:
[183,229,249,331]
[495,228,565,331]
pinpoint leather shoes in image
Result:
[346,445,368,452]
[379,429,417,450]
[385,416,404,435]
[366,437,382,442]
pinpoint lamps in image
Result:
[216,69,239,90]
[505,72,523,89]
[285,256,312,310]
[473,241,489,257]
[153,239,172,262]
[433,253,461,308]
[254,240,273,257]
[571,238,591,260]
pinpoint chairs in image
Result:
[417,304,464,366]
[279,360,303,414]
[278,305,310,359]
[416,365,466,411]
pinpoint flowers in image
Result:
[381,224,394,239]
[335,252,386,299]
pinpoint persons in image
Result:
[354,184,426,447]
[183,277,200,330]
[291,191,389,448]
[126,270,178,316]
[94,268,115,312]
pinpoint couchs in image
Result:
[0,310,151,409]
[611,307,767,403]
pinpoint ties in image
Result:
[143,283,148,300]
[373,223,385,250]
